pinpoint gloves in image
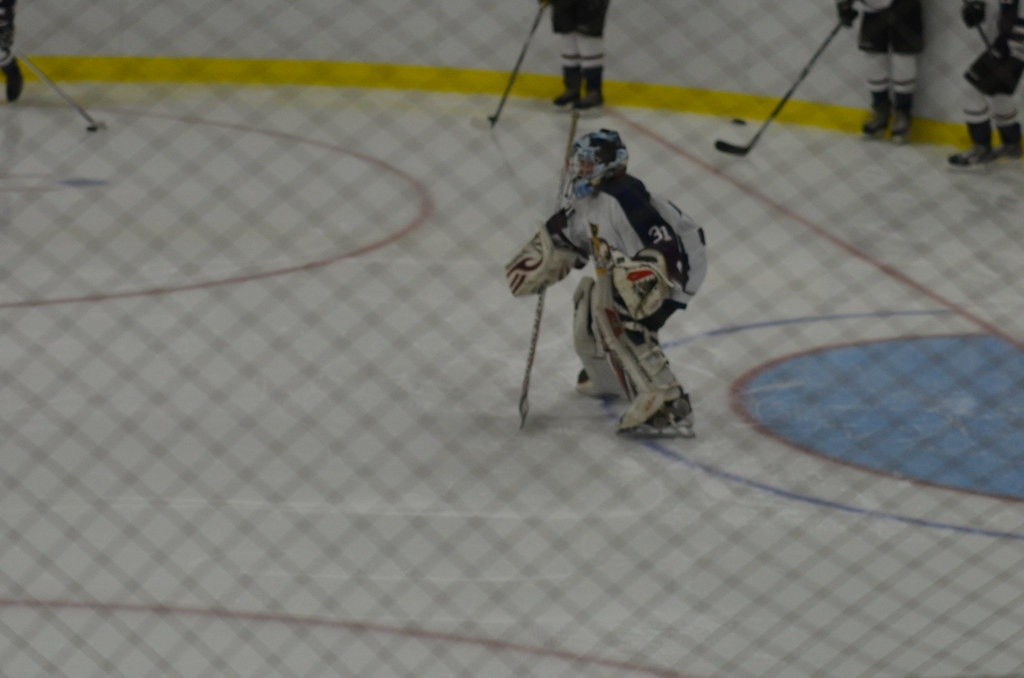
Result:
[994,57,1023,91]
[963,0,986,28]
[837,2,859,28]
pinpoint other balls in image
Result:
[86,125,99,132]
[733,118,746,125]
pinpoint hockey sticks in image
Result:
[714,22,842,158]
[517,110,581,431]
[468,3,547,132]
[8,44,107,129]
[974,25,992,49]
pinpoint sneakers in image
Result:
[572,90,604,115]
[862,98,892,137]
[576,369,600,397]
[630,394,696,437]
[990,143,1023,166]
[946,143,995,170]
[891,105,912,144]
[552,89,580,112]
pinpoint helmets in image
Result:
[562,128,629,201]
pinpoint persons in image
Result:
[948,0,1024,166]
[505,127,708,441]
[538,0,609,115]
[836,0,926,146]
[0,0,25,107]
